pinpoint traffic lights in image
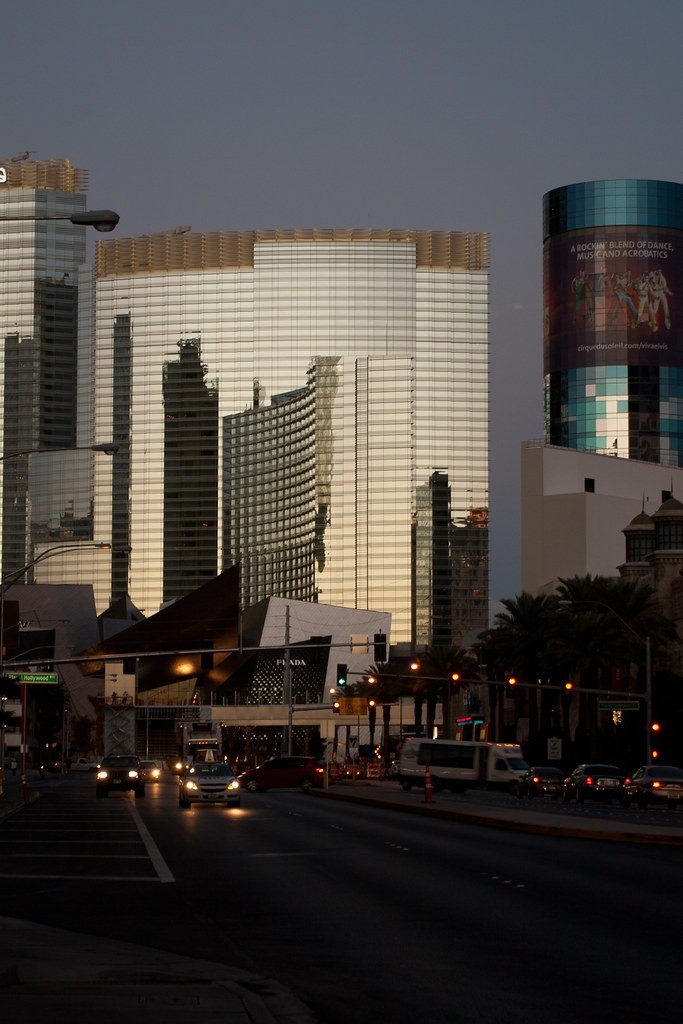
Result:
[333,702,340,713]
[652,747,663,762]
[367,696,377,711]
[651,719,664,736]
[505,673,515,697]
[564,680,575,704]
[337,664,347,686]
[450,671,460,695]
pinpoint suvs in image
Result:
[95,753,146,799]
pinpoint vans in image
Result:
[399,736,531,797]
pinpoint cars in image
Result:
[516,765,567,800]
[621,765,683,811]
[38,756,69,773]
[157,756,190,777]
[176,761,242,809]
[323,756,400,781]
[236,754,335,796]
[563,763,630,806]
[139,760,163,783]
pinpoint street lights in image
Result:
[558,600,652,765]
[0,542,112,803]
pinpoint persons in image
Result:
[66,757,72,774]
[122,691,128,705]
[11,759,18,777]
[236,758,245,774]
[111,692,117,705]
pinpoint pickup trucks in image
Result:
[68,757,101,773]
